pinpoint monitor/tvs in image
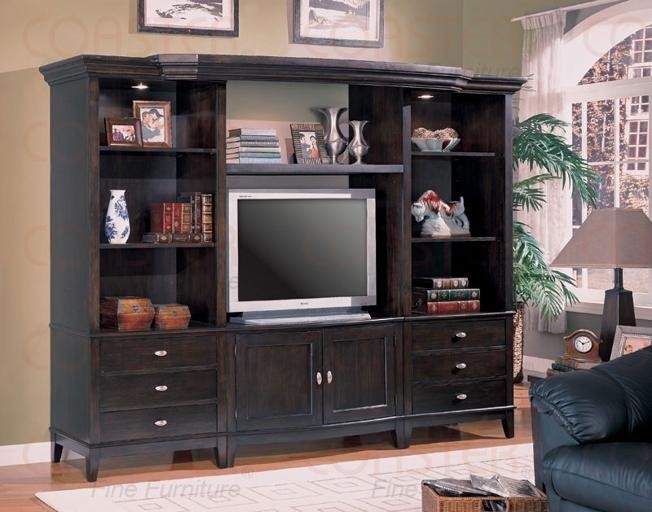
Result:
[226,188,377,324]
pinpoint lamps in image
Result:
[549,208,652,360]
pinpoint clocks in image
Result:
[558,330,603,364]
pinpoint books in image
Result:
[226,129,282,164]
[150,192,213,242]
[423,474,547,512]
[412,277,482,314]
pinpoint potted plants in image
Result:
[512,113,603,382]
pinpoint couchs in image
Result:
[528,345,652,512]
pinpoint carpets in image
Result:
[36,442,537,512]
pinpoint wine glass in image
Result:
[317,107,370,164]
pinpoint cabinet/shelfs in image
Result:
[38,54,528,482]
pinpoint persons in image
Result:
[625,345,634,354]
[113,110,164,142]
[299,134,312,159]
[310,136,320,159]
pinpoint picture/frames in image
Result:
[133,100,172,148]
[290,123,331,164]
[137,0,239,36]
[103,117,143,148]
[293,0,384,48]
[610,325,652,361]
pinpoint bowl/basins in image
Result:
[411,137,460,152]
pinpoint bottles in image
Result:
[105,189,130,245]
